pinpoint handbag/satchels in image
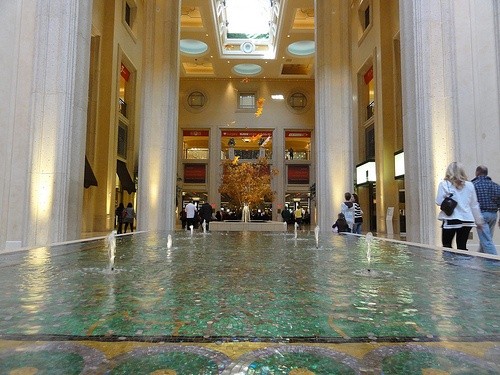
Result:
[122,208,127,218]
[440,196,458,216]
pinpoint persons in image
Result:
[470,165,499,255]
[435,161,484,251]
[115,203,125,234]
[351,194,363,234]
[179,199,310,232]
[123,203,136,233]
[340,192,355,232]
[332,213,351,233]
